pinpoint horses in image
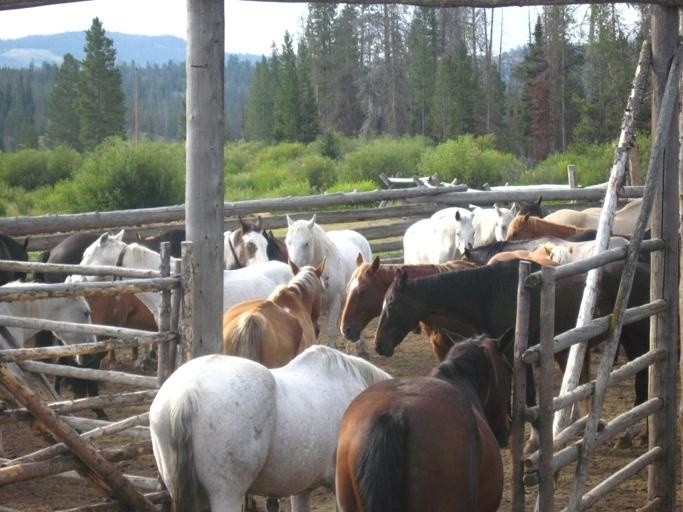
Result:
[0,215,376,421]
[344,198,678,447]
[149,331,518,512]
